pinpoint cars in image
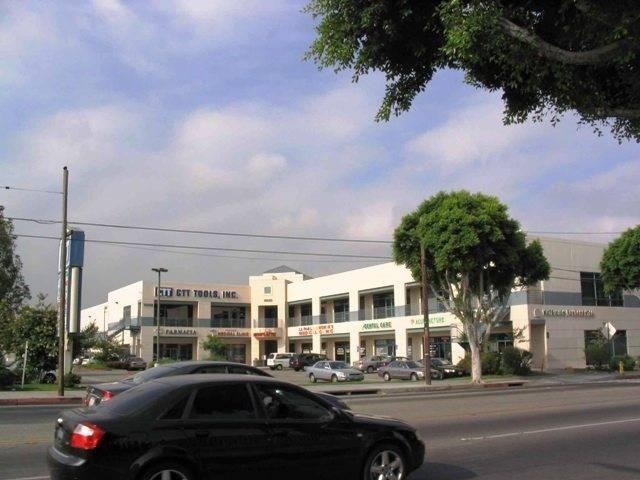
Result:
[352,355,388,373]
[417,357,463,379]
[46,373,425,480]
[303,361,365,384]
[377,359,440,382]
[379,356,411,368]
[84,359,351,414]
[4,352,56,385]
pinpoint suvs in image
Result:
[267,352,297,371]
[289,353,328,372]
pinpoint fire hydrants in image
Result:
[618,361,624,373]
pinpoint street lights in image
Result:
[151,268,168,367]
